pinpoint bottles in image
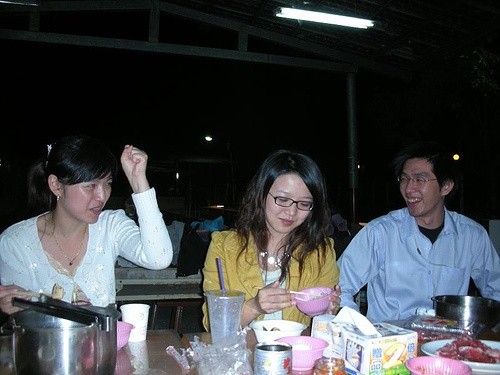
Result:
[312,357,347,375]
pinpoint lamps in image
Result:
[275,0,375,30]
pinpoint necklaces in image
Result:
[49,213,88,266]
[260,248,289,268]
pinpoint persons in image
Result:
[337,145,500,323]
[0,135,174,318]
[201,151,343,333]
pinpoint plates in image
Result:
[421,338,500,373]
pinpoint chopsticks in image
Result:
[253,286,323,301]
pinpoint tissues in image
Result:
[332,305,418,375]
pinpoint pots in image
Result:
[7,305,122,375]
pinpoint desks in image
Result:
[143,330,428,374]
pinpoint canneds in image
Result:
[313,357,346,375]
[254,340,293,374]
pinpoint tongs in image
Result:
[16,293,113,333]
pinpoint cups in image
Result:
[253,342,293,375]
[120,304,150,342]
[204,289,246,344]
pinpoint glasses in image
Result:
[268,192,314,211]
[397,175,438,186]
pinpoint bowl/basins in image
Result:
[116,320,133,350]
[293,286,334,316]
[249,319,305,343]
[275,335,329,371]
[405,355,473,375]
[431,295,500,330]
